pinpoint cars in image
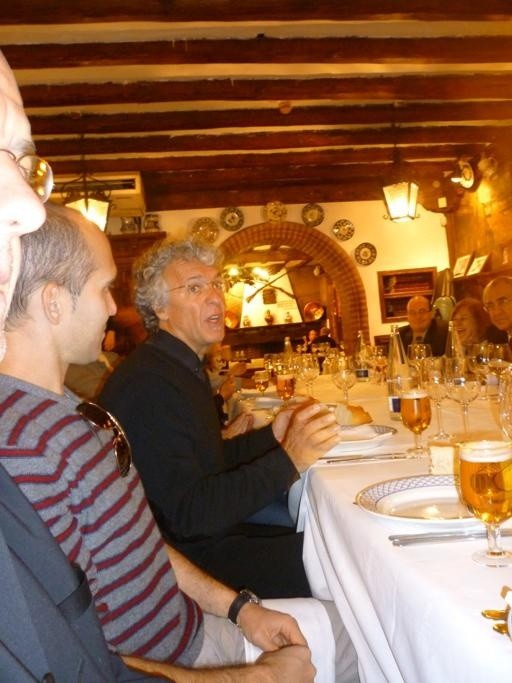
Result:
[220,345,232,360]
[235,350,248,362]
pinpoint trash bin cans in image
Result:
[227,587,262,625]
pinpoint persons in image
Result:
[396,294,448,356]
[98,236,341,599]
[0,199,360,683]
[451,296,493,345]
[211,362,261,441]
[306,326,336,352]
[307,329,319,345]
[0,51,180,683]
[482,274,512,350]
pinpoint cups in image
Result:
[164,280,226,298]
[0,148,54,202]
[76,402,131,477]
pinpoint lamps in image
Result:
[381,102,420,224]
[59,113,112,233]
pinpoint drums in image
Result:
[387,527,510,546]
[317,452,417,466]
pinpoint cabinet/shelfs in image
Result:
[243,314,249,326]
[388,325,415,421]
[264,309,273,325]
[283,311,292,323]
[284,337,292,362]
[444,321,464,383]
[354,331,373,384]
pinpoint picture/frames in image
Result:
[466,254,491,277]
[453,252,472,279]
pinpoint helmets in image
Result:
[304,301,324,322]
[301,203,324,228]
[333,218,355,241]
[354,242,378,266]
[193,217,219,246]
[225,311,238,331]
[220,207,244,231]
[264,200,287,225]
[357,474,484,525]
[460,162,476,189]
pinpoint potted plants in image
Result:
[466,254,491,277]
[453,252,472,279]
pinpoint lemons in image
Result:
[477,157,500,181]
[120,215,141,234]
[143,213,160,232]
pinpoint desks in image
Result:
[227,355,512,683]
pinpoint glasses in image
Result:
[59,113,112,233]
[381,102,420,224]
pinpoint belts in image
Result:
[454,439,512,568]
[251,341,512,454]
